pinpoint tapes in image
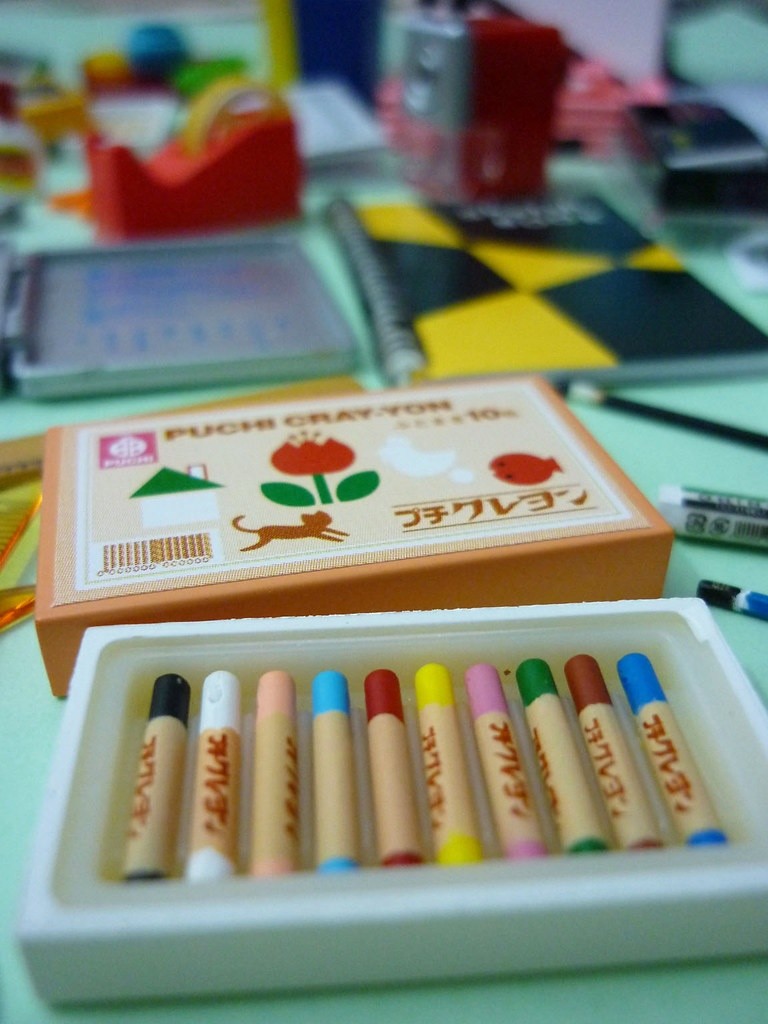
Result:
[182,76,291,153]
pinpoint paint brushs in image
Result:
[558,378,768,451]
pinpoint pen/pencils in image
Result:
[695,578,768,621]
